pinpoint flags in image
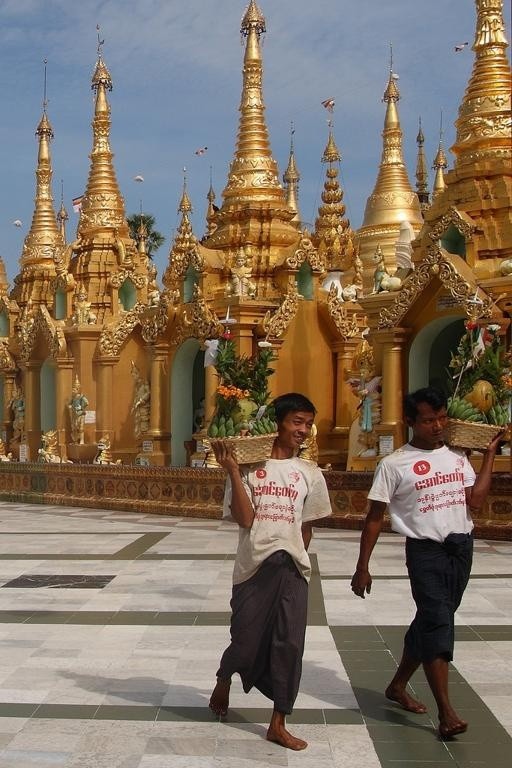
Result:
[321,98,335,113]
[71,195,84,213]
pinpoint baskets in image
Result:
[207,430,280,465]
[443,415,506,451]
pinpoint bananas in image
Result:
[445,396,510,426]
[207,413,279,438]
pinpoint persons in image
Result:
[350,386,511,737]
[210,392,332,751]
[129,358,150,440]
[194,397,205,433]
[344,365,382,459]
[6,378,24,443]
[66,373,89,443]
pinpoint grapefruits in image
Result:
[460,379,496,414]
[230,397,260,426]
